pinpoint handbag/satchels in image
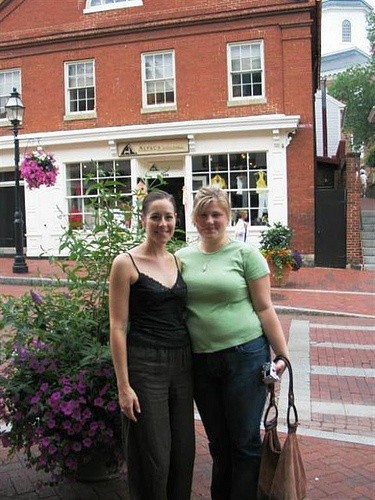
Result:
[256,353,307,500]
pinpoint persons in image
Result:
[172,184,290,500]
[234,211,249,243]
[109,191,198,500]
[356,167,368,198]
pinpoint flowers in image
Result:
[0,147,190,496]
[258,222,302,282]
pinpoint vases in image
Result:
[268,260,291,288]
[60,437,124,481]
